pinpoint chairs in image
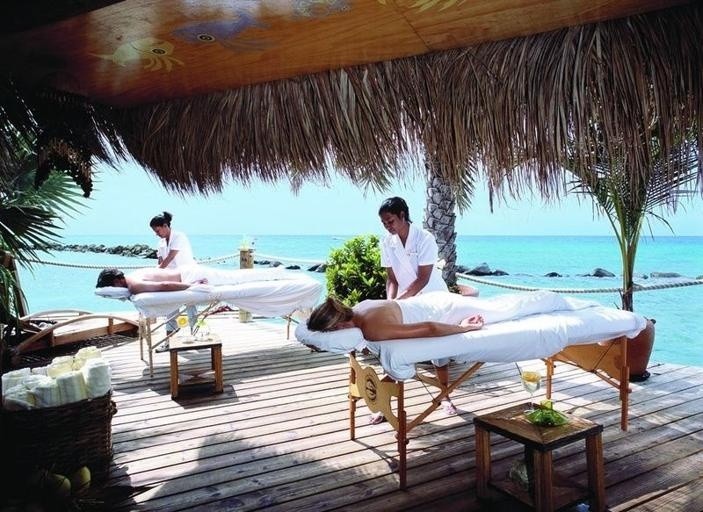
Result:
[0,308,142,356]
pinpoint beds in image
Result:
[131,270,321,378]
[295,296,646,491]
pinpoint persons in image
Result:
[366,195,452,426]
[94,262,210,293]
[304,289,576,342]
[148,211,211,355]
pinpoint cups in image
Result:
[522,370,542,415]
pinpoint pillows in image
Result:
[295,316,363,354]
[95,286,130,300]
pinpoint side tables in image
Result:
[473,402,608,511]
[168,332,223,399]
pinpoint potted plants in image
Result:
[560,105,699,379]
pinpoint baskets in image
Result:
[1,389,117,486]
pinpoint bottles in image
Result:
[192,317,211,341]
